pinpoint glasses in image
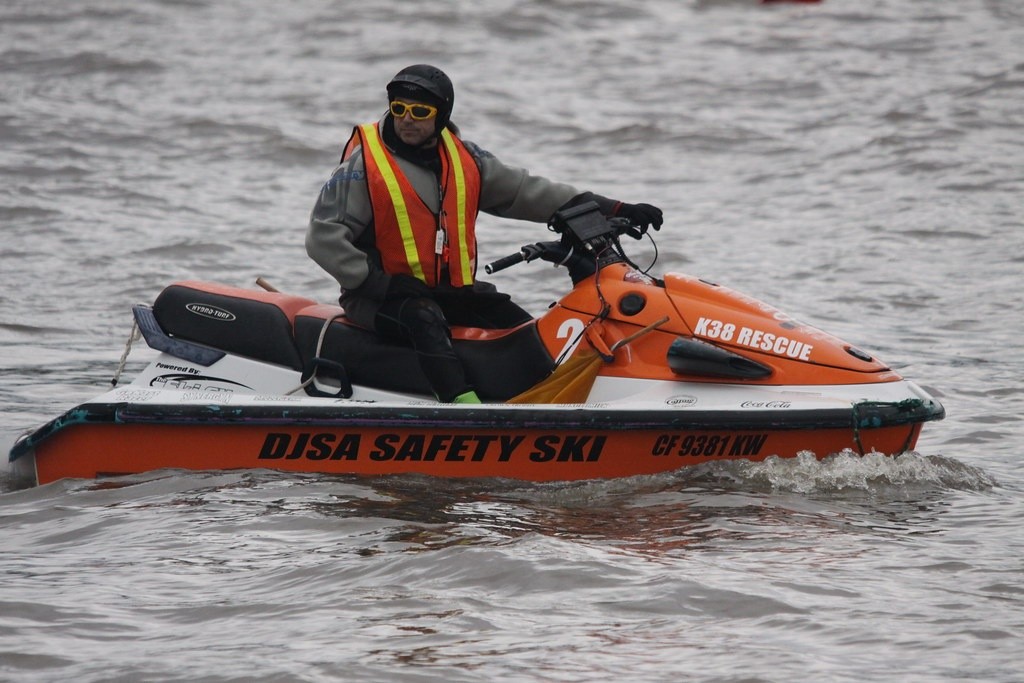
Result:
[389,102,438,121]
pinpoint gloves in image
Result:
[615,202,664,240]
[386,273,433,300]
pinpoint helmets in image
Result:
[386,65,454,112]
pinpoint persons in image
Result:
[305,63,664,405]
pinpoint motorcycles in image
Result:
[10,198,945,492]
